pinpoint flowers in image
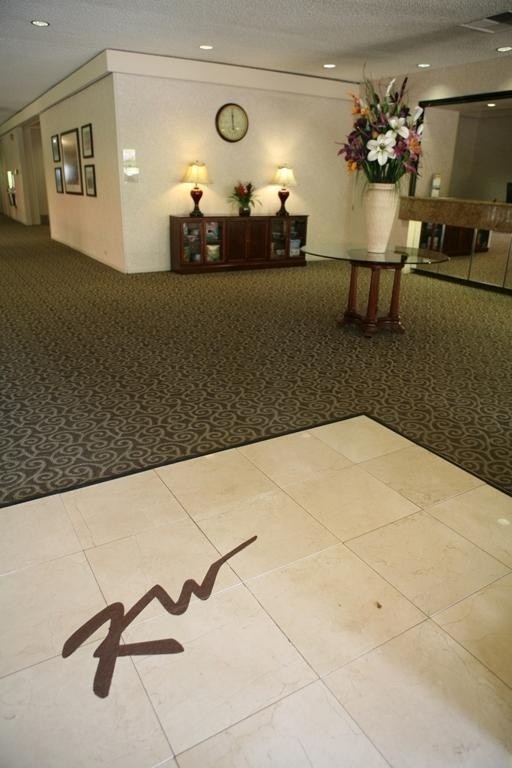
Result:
[335,62,424,184]
[226,181,263,209]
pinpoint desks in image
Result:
[398,196,512,290]
[302,244,450,338]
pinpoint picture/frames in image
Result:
[59,128,83,196]
[51,134,60,162]
[80,123,94,158]
[84,164,97,197]
[54,167,64,193]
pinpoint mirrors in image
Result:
[404,89,511,295]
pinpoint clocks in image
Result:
[215,102,249,143]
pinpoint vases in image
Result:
[363,182,401,255]
[239,204,251,216]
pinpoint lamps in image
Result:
[270,167,298,216]
[180,160,214,217]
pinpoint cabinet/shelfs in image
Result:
[170,215,307,274]
[423,222,491,258]
